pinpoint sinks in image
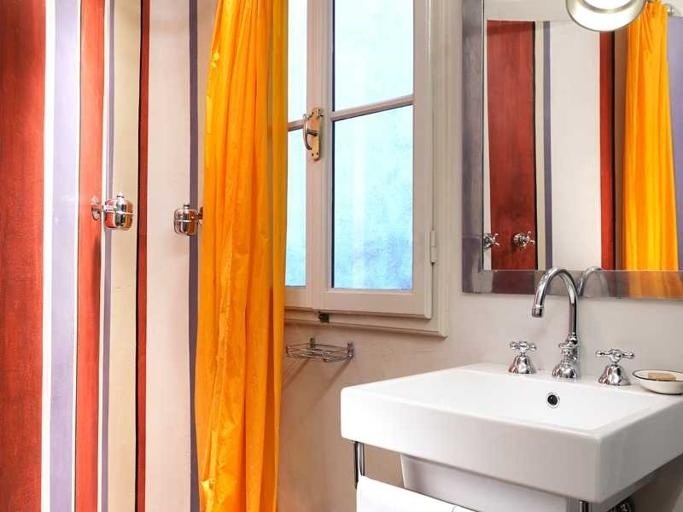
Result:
[339,362,682,503]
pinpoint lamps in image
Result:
[565,0,648,32]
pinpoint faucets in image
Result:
[531,266,577,339]
[577,266,611,298]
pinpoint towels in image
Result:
[356,475,479,512]
